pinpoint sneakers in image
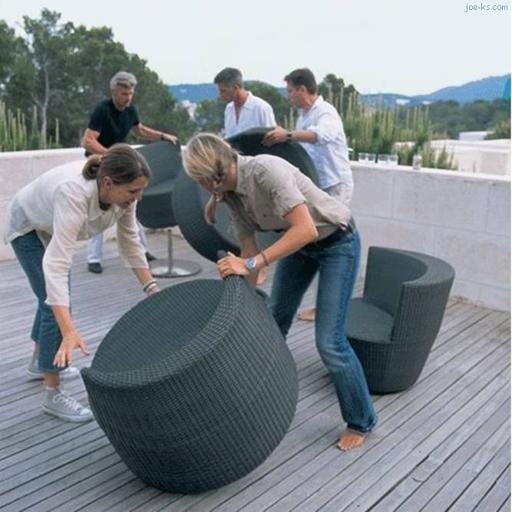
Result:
[40,387,94,422]
[26,360,80,380]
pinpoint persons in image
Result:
[0,139,161,423]
[211,66,279,141]
[77,68,183,275]
[259,68,356,324]
[178,130,380,452]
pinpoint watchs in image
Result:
[243,256,260,277]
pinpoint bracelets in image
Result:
[286,128,292,142]
[258,250,270,267]
[160,127,166,143]
[141,278,158,294]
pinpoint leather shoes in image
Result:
[88,263,102,273]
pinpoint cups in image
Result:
[359,152,399,165]
[348,148,354,162]
[411,150,422,170]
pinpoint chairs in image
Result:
[79,250,297,494]
[344,246,454,394]
[169,127,322,263]
[128,139,201,279]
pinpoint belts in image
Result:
[304,218,355,248]
